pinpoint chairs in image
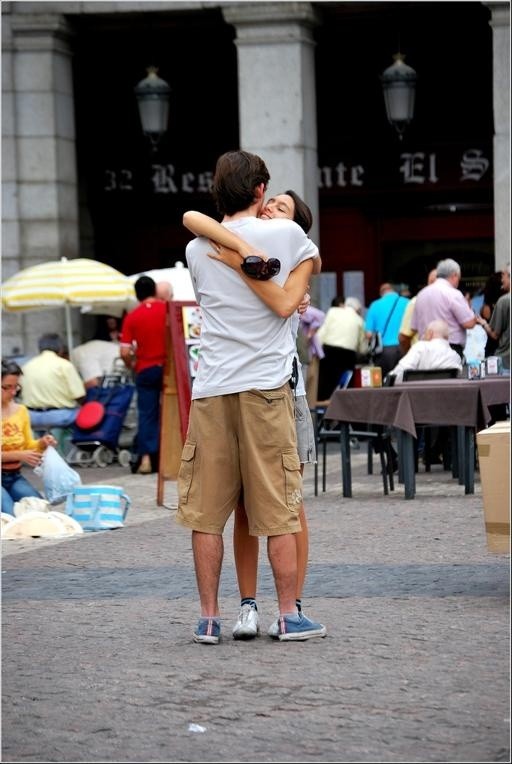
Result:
[382,369,459,491]
[310,369,396,497]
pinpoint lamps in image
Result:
[132,66,174,152]
[379,52,418,143]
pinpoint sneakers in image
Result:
[193,618,221,644]
[232,604,327,641]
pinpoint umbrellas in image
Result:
[0,256,136,356]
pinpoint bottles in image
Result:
[467,357,486,380]
[354,361,384,388]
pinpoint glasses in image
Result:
[240,255,282,280]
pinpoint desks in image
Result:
[322,375,510,500]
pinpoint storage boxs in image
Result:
[475,421,511,557]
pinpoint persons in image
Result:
[181,190,317,637]
[174,151,327,643]
[0,360,57,517]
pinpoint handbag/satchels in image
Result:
[370,332,383,356]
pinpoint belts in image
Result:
[27,406,57,412]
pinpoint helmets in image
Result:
[2,383,23,393]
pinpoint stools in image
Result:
[31,425,76,434]
